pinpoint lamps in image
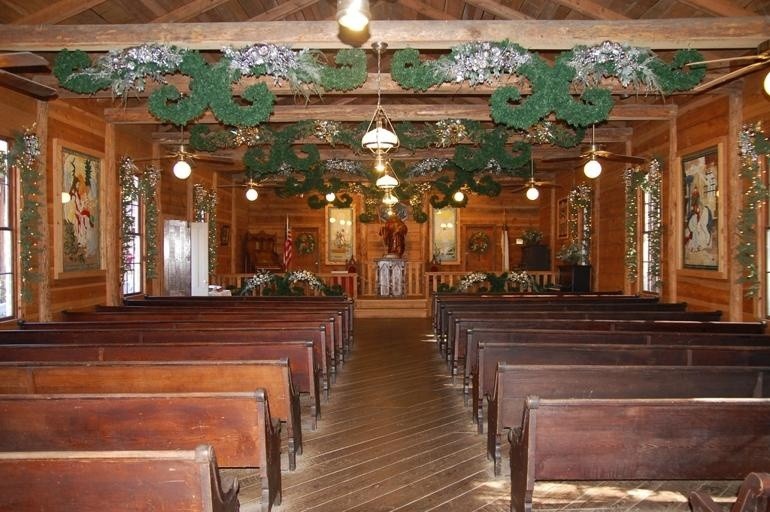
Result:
[525,184,541,201]
[244,184,259,203]
[583,152,603,179]
[336,0,402,218]
[172,153,194,180]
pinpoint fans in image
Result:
[536,123,646,166]
[112,124,235,166]
[210,168,288,190]
[493,150,563,192]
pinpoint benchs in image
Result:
[2,292,356,510]
[428,289,770,510]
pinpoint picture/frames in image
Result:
[676,135,728,281]
[51,137,107,280]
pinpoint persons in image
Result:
[688,202,711,251]
[67,177,97,245]
[336,228,346,246]
